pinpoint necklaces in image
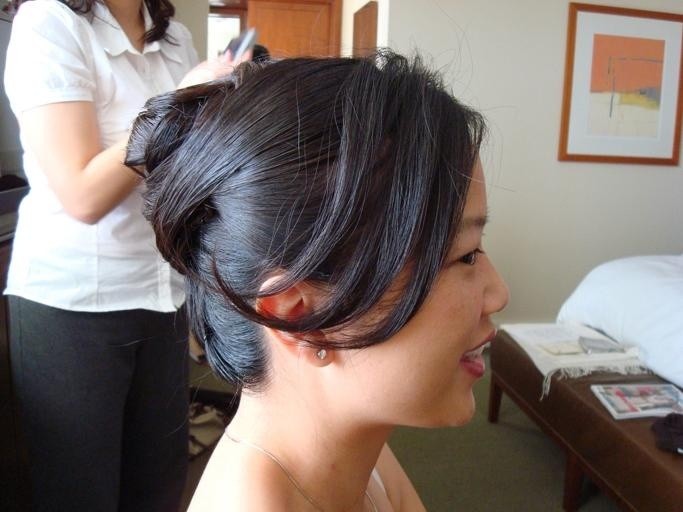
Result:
[225,427,378,512]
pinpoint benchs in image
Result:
[487,323,683,512]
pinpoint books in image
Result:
[592,383,683,422]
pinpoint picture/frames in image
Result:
[556,0,682,167]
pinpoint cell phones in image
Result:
[234,26,258,61]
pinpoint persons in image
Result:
[228,39,270,64]
[123,44,510,512]
[2,0,254,512]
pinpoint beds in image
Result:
[556,255,683,391]
